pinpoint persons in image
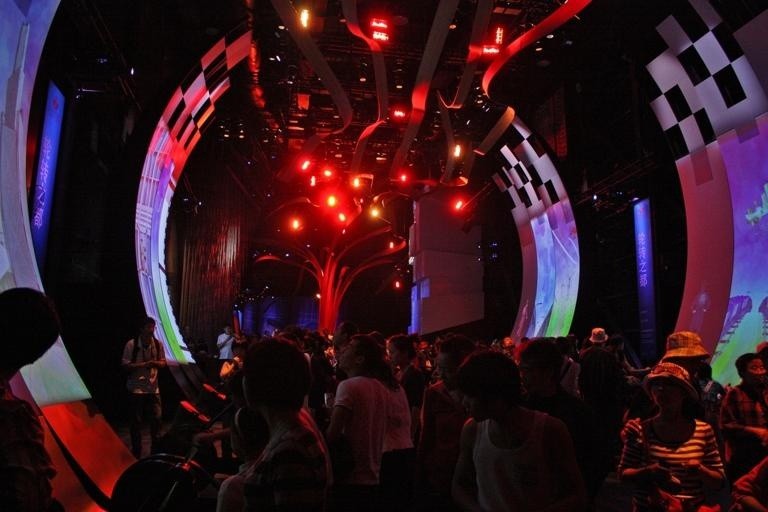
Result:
[2,320,767,511]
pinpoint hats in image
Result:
[589,327,608,342]
[643,362,699,399]
[661,332,711,359]
